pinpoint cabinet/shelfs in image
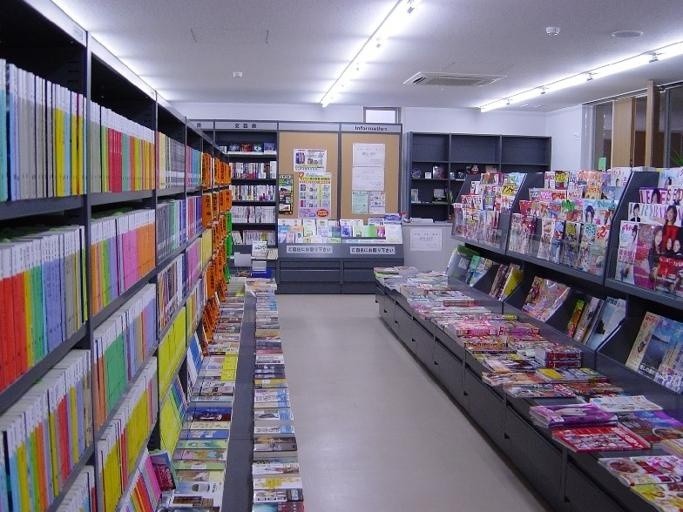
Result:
[207,118,275,247]
[339,122,402,221]
[375,266,682,512]
[402,131,552,223]
[277,121,338,220]
[153,278,306,512]
[446,165,681,402]
[406,218,462,274]
[0,0,232,512]
[275,216,405,294]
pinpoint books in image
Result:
[411,165,497,205]
[530,395,682,511]
[375,266,492,319]
[158,130,231,456]
[278,212,400,244]
[454,171,528,302]
[90,100,177,512]
[171,277,304,512]
[0,60,97,512]
[436,313,623,398]
[508,168,643,350]
[615,168,682,393]
[222,143,277,272]
